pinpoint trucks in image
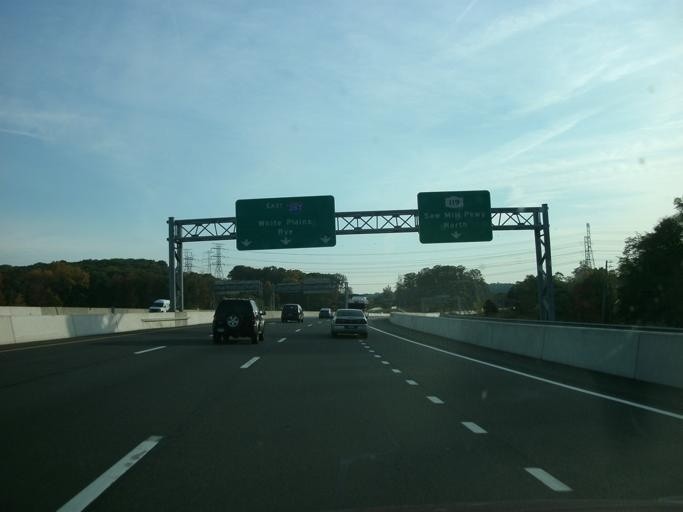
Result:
[348,295,370,317]
[147,298,170,313]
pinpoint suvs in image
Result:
[278,302,304,323]
[212,298,264,344]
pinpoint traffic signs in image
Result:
[416,190,494,244]
[233,195,337,251]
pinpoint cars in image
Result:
[317,308,332,319]
[329,310,369,339]
